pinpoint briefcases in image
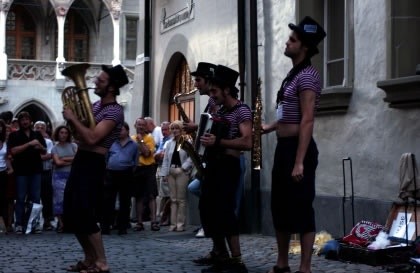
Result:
[339,201,420,266]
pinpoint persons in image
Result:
[101,116,246,238]
[57,63,129,273]
[261,15,327,273]
[0,110,79,235]
[171,62,254,273]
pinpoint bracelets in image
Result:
[213,136,221,145]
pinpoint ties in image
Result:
[275,58,311,109]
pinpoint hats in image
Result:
[206,65,240,88]
[101,64,129,94]
[288,15,327,54]
[12,118,19,124]
[189,62,217,79]
[34,121,46,127]
[19,112,28,118]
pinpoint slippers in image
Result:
[80,263,110,273]
[68,260,89,272]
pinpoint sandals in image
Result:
[133,223,144,232]
[151,221,160,230]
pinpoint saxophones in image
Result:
[173,88,206,180]
[251,77,264,170]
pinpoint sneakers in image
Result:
[15,225,23,235]
[33,230,43,234]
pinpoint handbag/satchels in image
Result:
[26,203,44,234]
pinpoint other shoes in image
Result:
[6,226,13,233]
[118,229,127,235]
[270,265,290,273]
[57,224,62,233]
[195,227,206,237]
[193,251,229,266]
[100,230,112,235]
[200,261,249,273]
[44,224,56,230]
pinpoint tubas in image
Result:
[61,63,98,130]
